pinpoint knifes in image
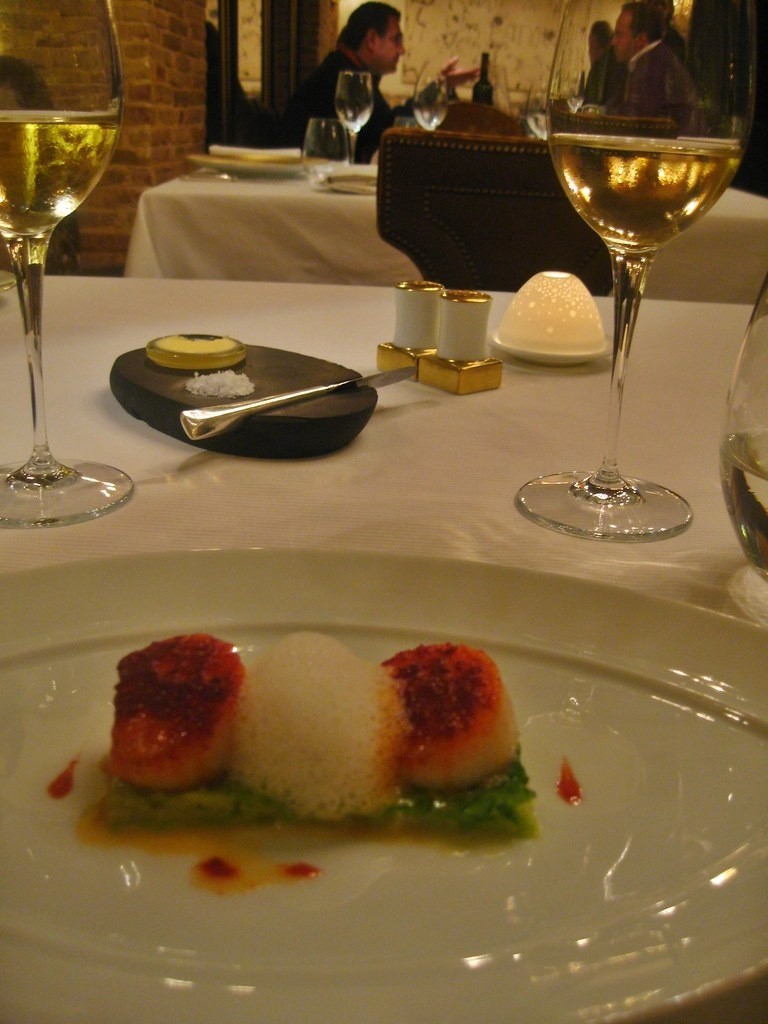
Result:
[180,366,419,441]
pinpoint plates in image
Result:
[0,551,768,1024]
[187,153,303,177]
[487,331,607,364]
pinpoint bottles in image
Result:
[472,52,493,105]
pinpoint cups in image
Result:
[526,82,547,140]
[301,118,349,182]
[719,268,768,584]
[413,74,447,129]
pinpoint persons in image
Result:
[0,54,82,275]
[277,2,478,166]
[206,21,246,149]
[583,0,710,138]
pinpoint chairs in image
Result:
[551,97,678,141]
[437,101,526,136]
[377,129,613,296]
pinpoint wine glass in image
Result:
[514,1,750,541]
[334,72,373,170]
[0,0,135,528]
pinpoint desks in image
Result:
[0,276,767,624]
[123,163,767,307]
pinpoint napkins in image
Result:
[208,145,303,162]
[323,164,376,194]
